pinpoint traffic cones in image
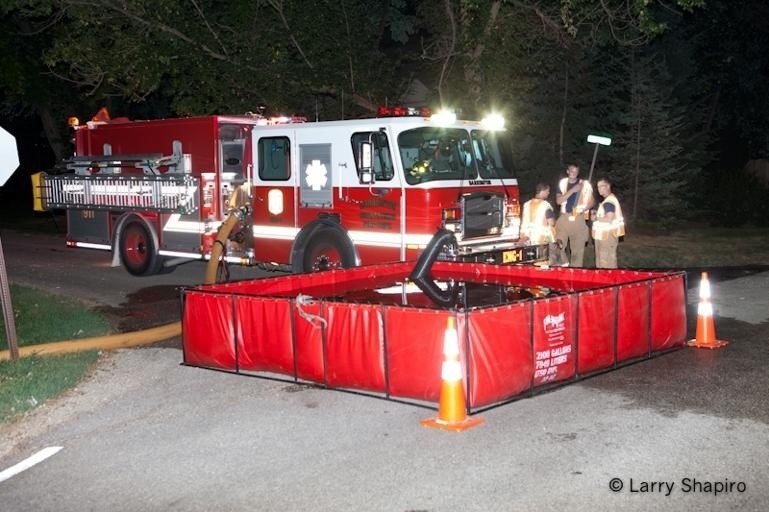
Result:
[681,265,734,351]
[416,315,487,435]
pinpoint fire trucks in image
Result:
[28,93,526,284]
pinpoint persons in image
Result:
[550,162,595,268]
[516,182,565,268]
[587,176,628,270]
